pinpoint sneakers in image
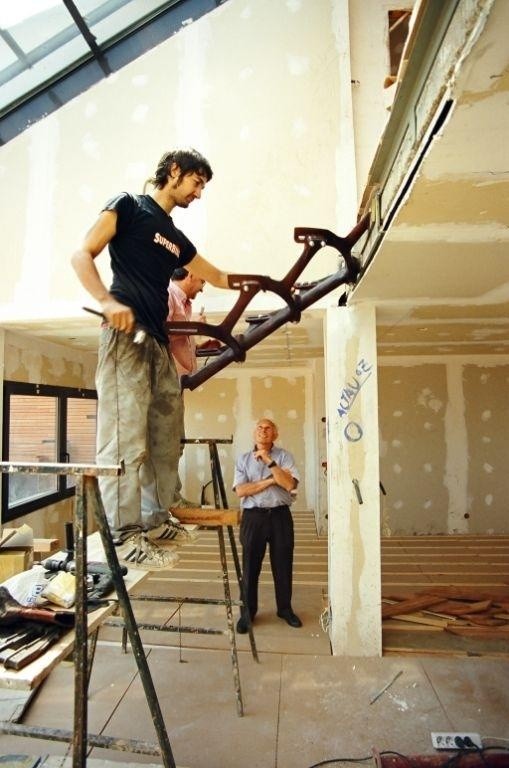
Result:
[171,499,200,508]
[168,511,180,526]
[142,521,200,545]
[115,532,180,571]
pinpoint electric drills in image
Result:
[32,522,128,601]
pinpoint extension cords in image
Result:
[431,732,483,750]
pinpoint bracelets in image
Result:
[268,460,277,468]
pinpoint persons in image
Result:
[231,418,303,634]
[164,265,207,526]
[69,144,301,571]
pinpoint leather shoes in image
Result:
[277,611,301,627]
[237,616,254,634]
